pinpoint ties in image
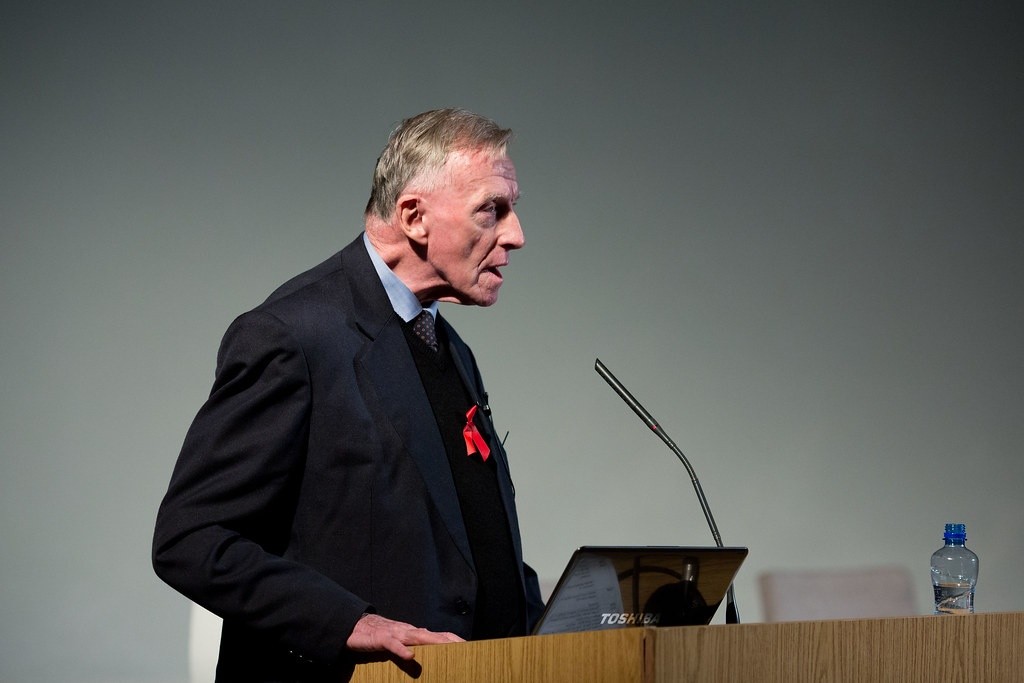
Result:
[411,310,437,352]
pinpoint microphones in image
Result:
[593,358,740,623]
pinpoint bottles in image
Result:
[930,524,979,615]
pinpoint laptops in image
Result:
[531,547,748,636]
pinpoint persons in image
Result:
[152,108,547,683]
